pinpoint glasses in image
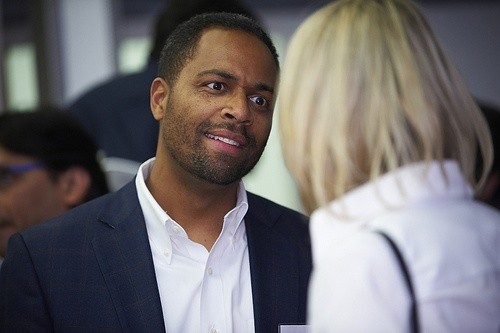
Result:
[0,163,42,193]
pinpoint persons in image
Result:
[0,105,110,264]
[278,0,500,333]
[0,12,313,333]
